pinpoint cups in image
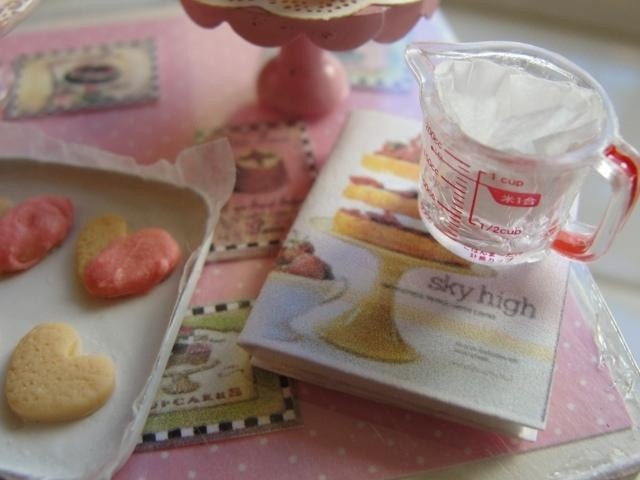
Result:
[404,40,640,267]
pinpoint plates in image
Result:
[182,0,436,122]
[0,152,218,477]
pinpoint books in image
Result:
[232,105,582,445]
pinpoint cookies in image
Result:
[5,323,116,426]
[75,214,180,301]
[1,194,74,275]
